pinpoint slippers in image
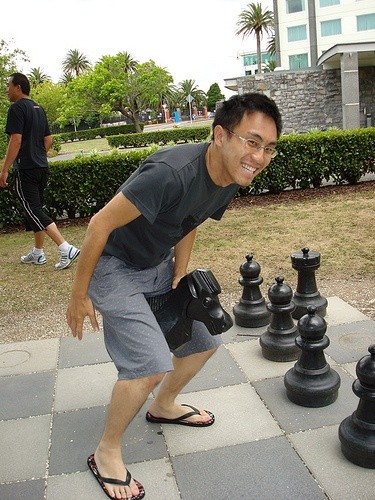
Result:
[87,453,146,500]
[145,404,214,427]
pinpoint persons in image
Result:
[65,91,282,500]
[174,109,180,123]
[0,73,80,269]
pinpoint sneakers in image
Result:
[55,245,80,270]
[20,249,46,265]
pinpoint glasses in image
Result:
[224,127,279,160]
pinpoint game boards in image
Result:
[0,295,375,500]
